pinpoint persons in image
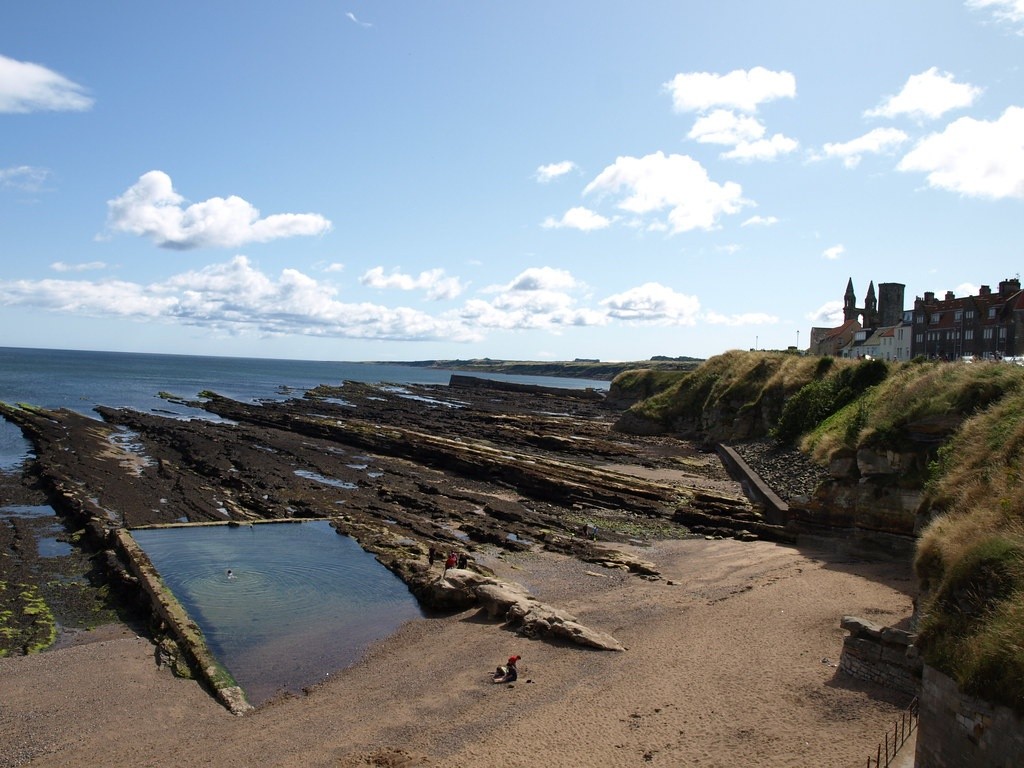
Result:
[427,543,468,572]
[582,522,599,543]
[488,655,522,684]
